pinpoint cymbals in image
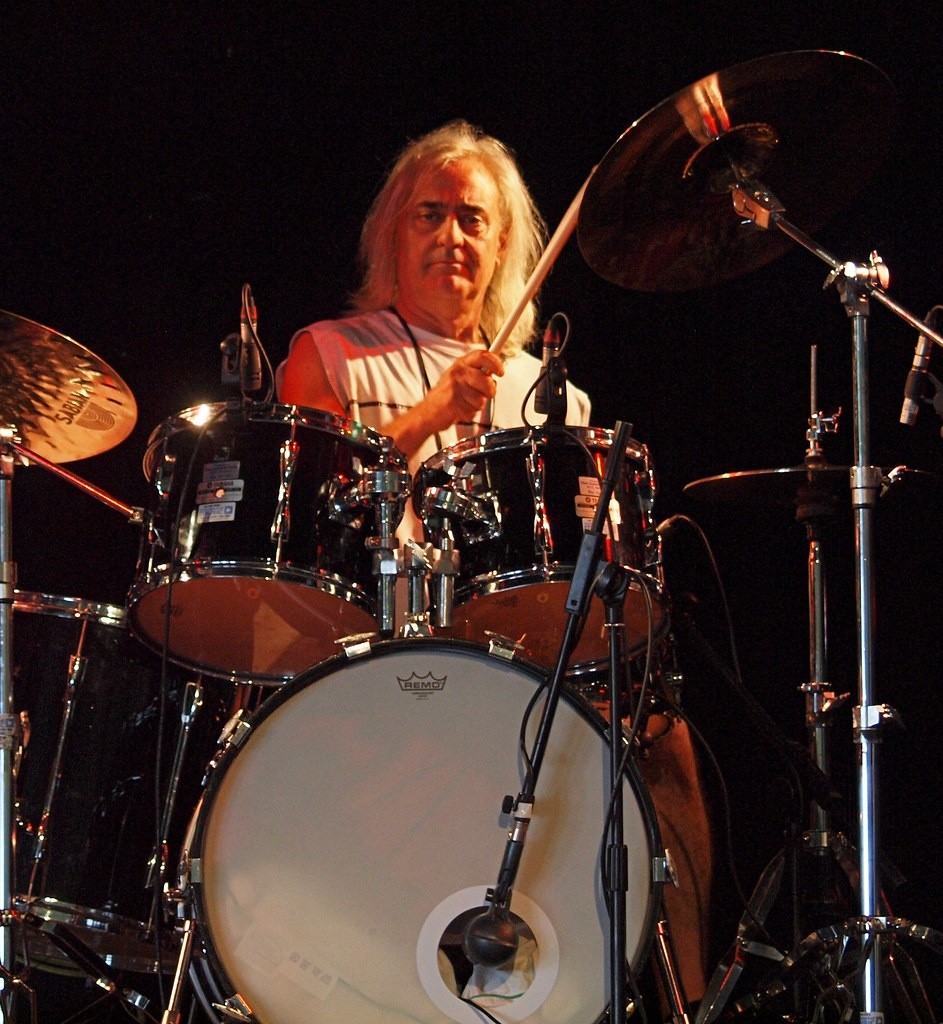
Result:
[0,306,138,466]
[684,460,938,515]
[577,48,900,295]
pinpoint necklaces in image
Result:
[387,304,490,450]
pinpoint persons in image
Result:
[276,118,740,1024]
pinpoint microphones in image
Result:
[535,323,566,416]
[900,309,936,427]
[462,909,519,968]
[239,286,261,389]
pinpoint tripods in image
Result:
[692,152,943,1024]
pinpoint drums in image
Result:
[412,425,672,681]
[176,634,675,1024]
[10,587,243,982]
[127,396,408,691]
